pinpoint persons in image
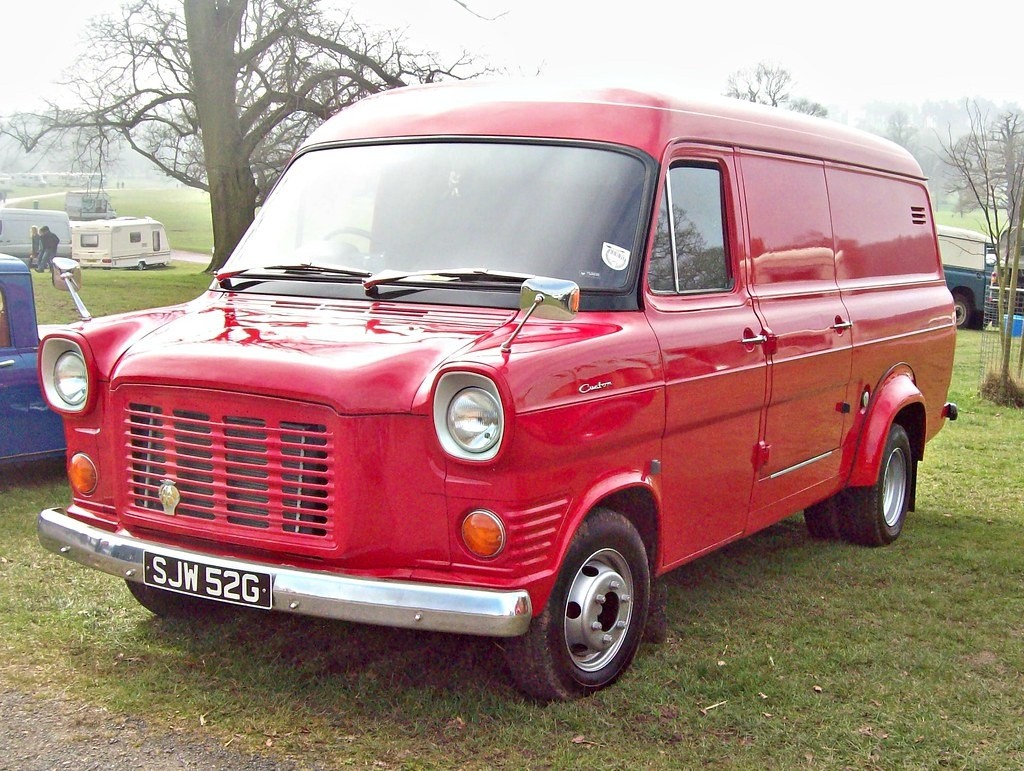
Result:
[28,226,60,273]
[0,192,7,203]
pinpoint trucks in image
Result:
[68,216,170,271]
[0,208,71,266]
[64,190,117,220]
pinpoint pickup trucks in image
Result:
[0,253,68,464]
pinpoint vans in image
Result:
[36,71,960,701]
[987,219,1024,326]
[742,245,855,370]
[0,172,108,187]
[935,226,998,329]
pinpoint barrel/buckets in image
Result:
[1003,315,1024,337]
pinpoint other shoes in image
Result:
[35,269,44,273]
